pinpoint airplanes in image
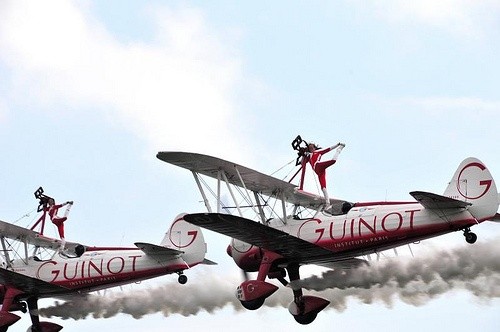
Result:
[156,135,500,325]
[0,186,217,332]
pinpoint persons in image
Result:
[42,197,74,252]
[300,142,346,211]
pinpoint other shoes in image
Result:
[324,202,331,211]
[336,144,345,153]
[59,245,64,250]
[66,204,71,211]
